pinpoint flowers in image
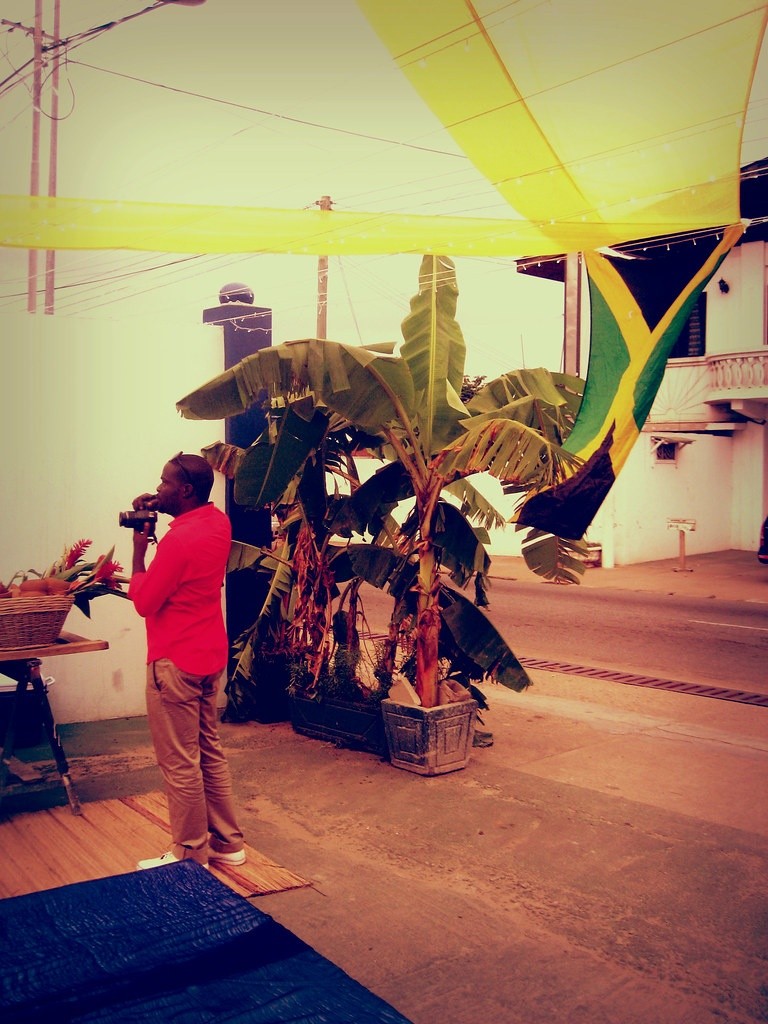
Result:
[40,538,132,621]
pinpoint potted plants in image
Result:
[175,255,604,776]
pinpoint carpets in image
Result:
[0,857,414,1024]
[0,788,313,898]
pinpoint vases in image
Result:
[0,595,75,650]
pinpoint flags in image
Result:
[507,225,743,541]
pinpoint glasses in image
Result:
[169,451,190,482]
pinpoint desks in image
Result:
[0,630,111,815]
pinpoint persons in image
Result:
[129,452,246,871]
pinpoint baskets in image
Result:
[0,595,76,650]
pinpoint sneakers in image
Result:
[207,843,246,865]
[136,851,209,871]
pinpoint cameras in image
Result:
[118,507,158,536]
